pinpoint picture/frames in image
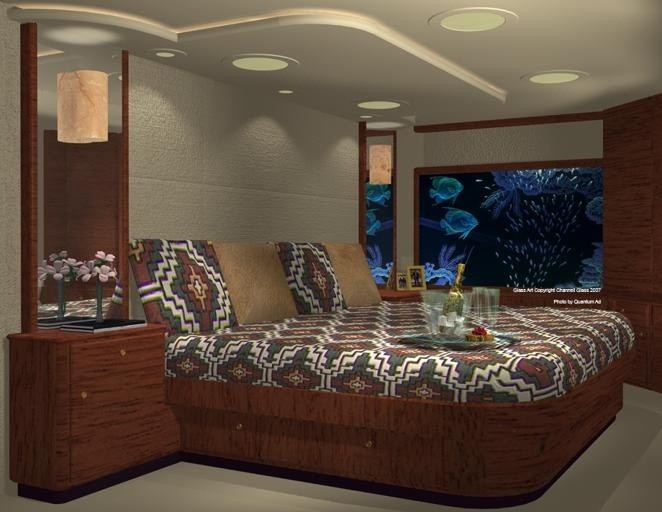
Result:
[396,264,427,292]
[363,169,394,289]
[414,158,603,299]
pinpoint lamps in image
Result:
[369,144,391,185]
[55,68,110,143]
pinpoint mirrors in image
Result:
[360,121,398,290]
[19,22,131,333]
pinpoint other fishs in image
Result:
[365,208,381,236]
[366,181,391,205]
[475,167,593,290]
[430,176,464,205]
[439,207,480,240]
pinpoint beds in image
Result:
[167,299,636,499]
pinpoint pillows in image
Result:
[128,238,383,334]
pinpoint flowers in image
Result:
[35,250,80,319]
[75,249,119,322]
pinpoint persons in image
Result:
[412,269,421,287]
[399,276,406,288]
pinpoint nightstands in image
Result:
[379,290,422,303]
[8,325,174,491]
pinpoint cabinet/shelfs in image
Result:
[600,94,662,392]
